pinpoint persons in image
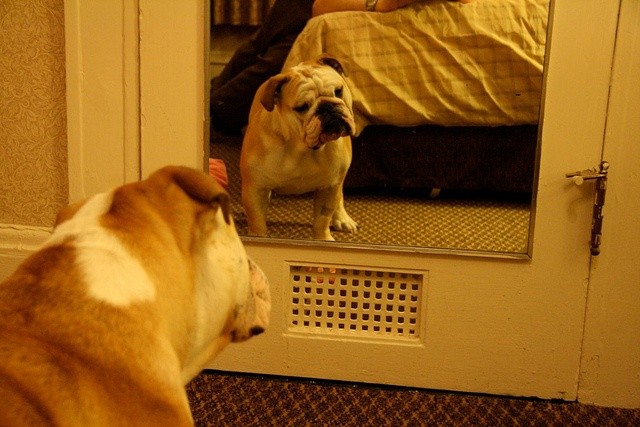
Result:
[210,0,409,133]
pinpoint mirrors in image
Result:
[207,0,557,265]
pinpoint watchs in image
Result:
[366,0,377,11]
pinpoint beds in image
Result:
[282,0,552,204]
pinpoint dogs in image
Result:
[1,163,272,426]
[239,53,357,240]
